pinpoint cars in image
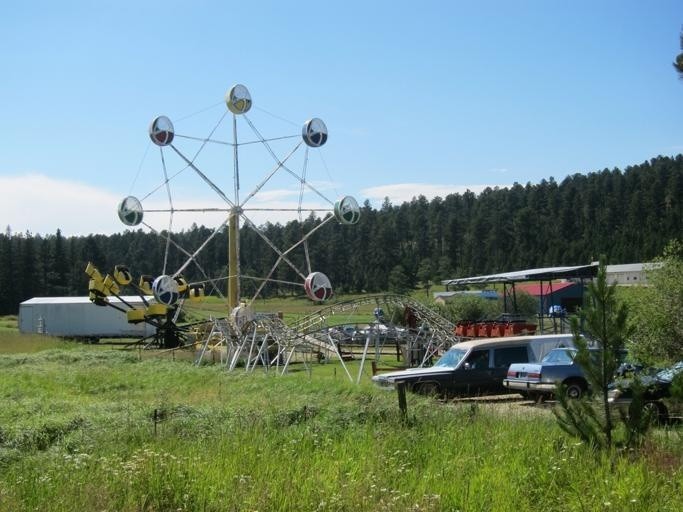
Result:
[498,345,630,402]
[310,322,447,347]
[546,305,565,317]
[603,363,682,429]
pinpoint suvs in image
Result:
[365,332,587,405]
